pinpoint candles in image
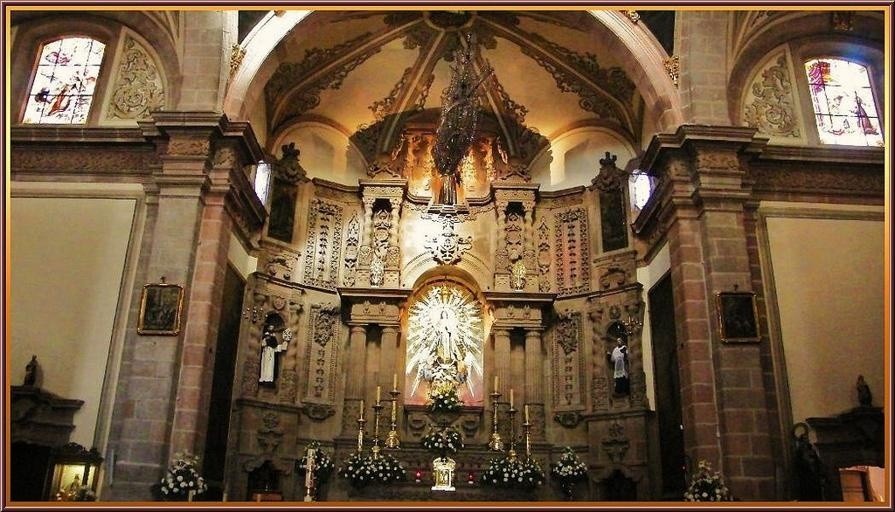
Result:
[494,374,529,420]
[358,372,398,414]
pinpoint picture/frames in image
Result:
[135,281,185,337]
[714,289,765,346]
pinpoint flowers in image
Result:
[293,438,405,487]
[681,458,734,502]
[422,381,465,456]
[480,443,590,489]
[158,448,211,500]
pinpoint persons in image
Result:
[606,337,628,394]
[262,323,279,382]
